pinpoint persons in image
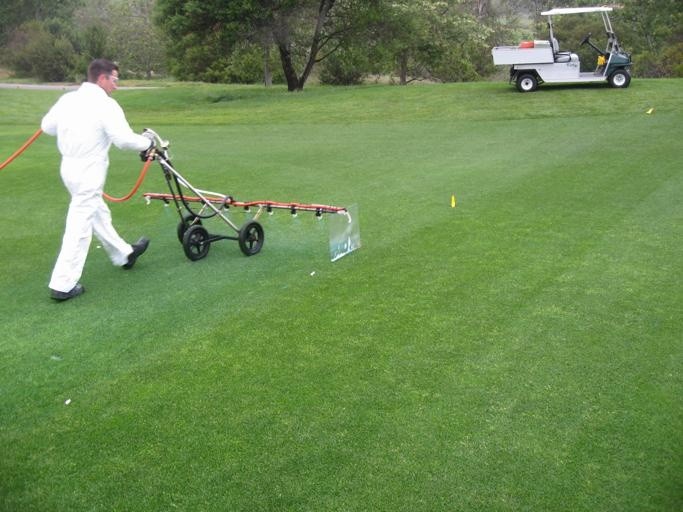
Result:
[38,58,155,300]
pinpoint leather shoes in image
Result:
[51,283,85,301]
[123,236,149,269]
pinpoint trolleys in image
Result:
[135,125,265,262]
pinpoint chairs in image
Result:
[553,36,579,62]
